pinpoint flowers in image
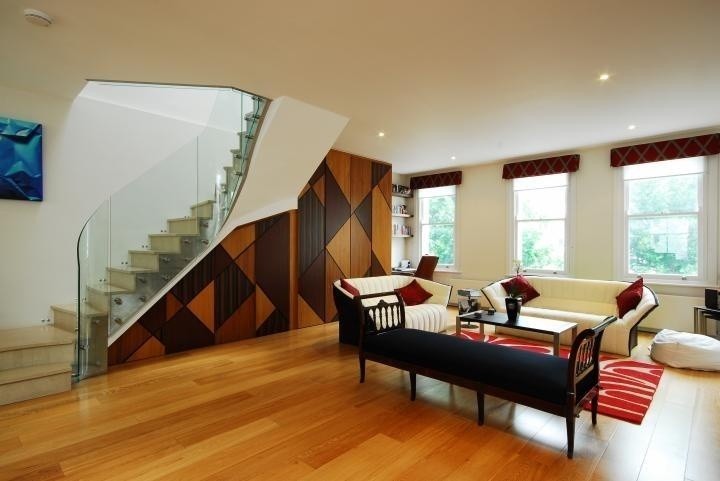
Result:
[508,259,523,299]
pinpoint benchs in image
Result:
[355,292,619,461]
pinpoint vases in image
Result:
[505,298,522,325]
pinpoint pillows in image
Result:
[616,278,644,319]
[394,279,433,306]
[501,276,540,306]
[340,278,360,298]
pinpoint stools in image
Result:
[650,329,720,372]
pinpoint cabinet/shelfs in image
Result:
[392,192,415,238]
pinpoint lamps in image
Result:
[23,9,52,27]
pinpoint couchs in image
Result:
[481,276,660,358]
[333,275,453,348]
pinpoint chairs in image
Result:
[414,256,439,280]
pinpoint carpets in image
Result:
[450,330,665,426]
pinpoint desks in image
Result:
[456,289,482,329]
[693,306,720,342]
[392,268,417,275]
